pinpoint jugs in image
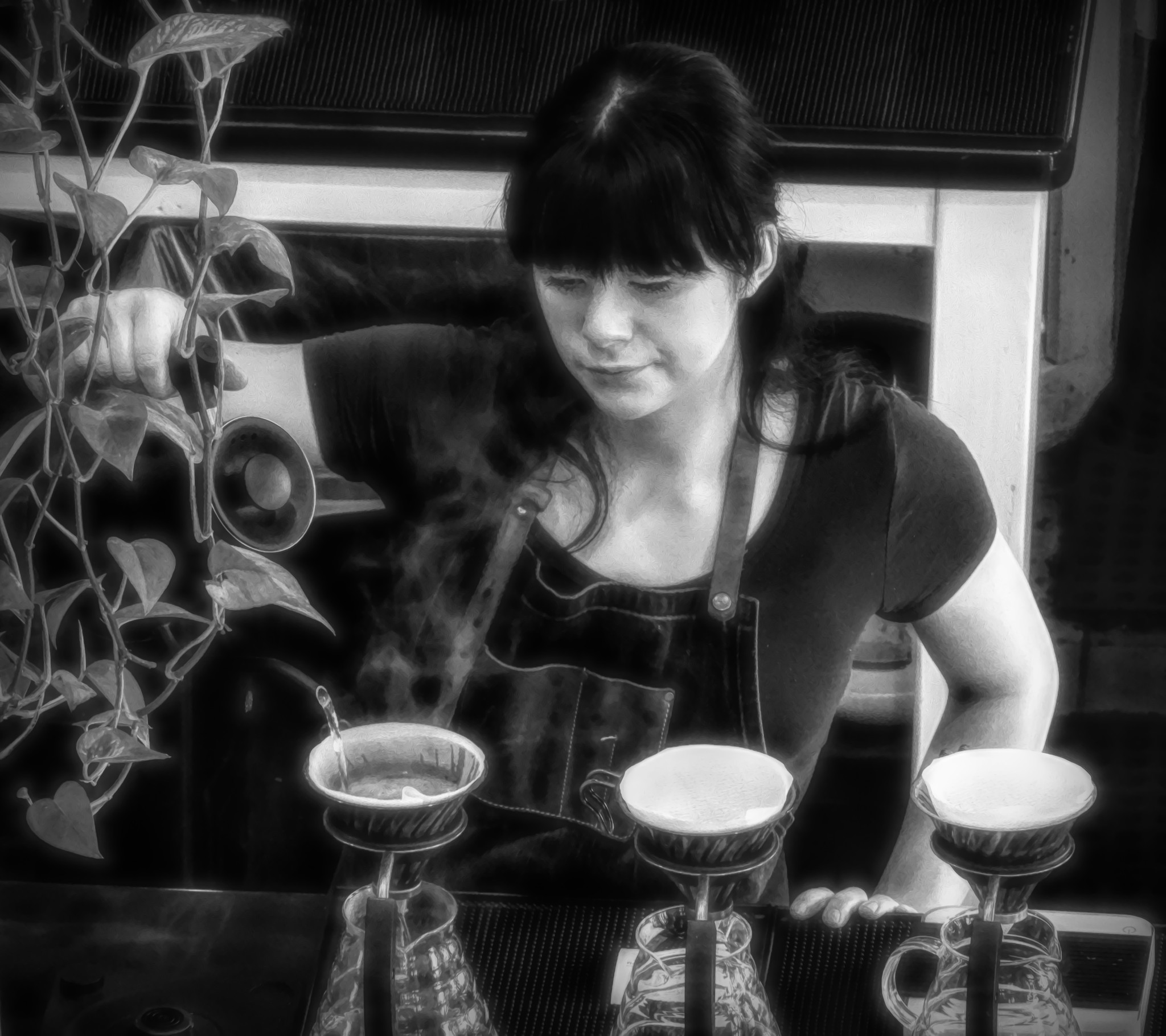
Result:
[611,900,776,1036]
[304,877,496,1035]
[880,907,1083,1035]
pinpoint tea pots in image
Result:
[9,333,335,712]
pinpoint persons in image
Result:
[23,43,1060,931]
